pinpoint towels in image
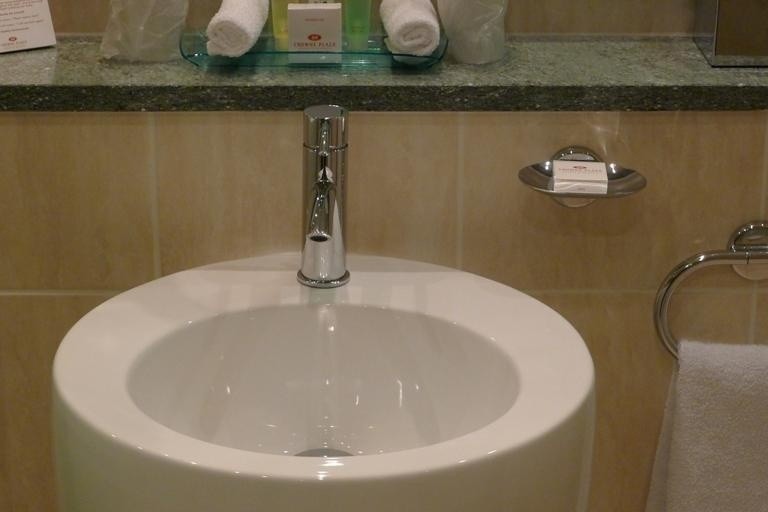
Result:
[205,0,270,57]
[644,340,768,512]
[379,0,441,57]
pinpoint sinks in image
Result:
[51,253,596,511]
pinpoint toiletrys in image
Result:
[270,0,298,51]
[344,0,373,51]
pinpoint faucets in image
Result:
[298,105,353,289]
